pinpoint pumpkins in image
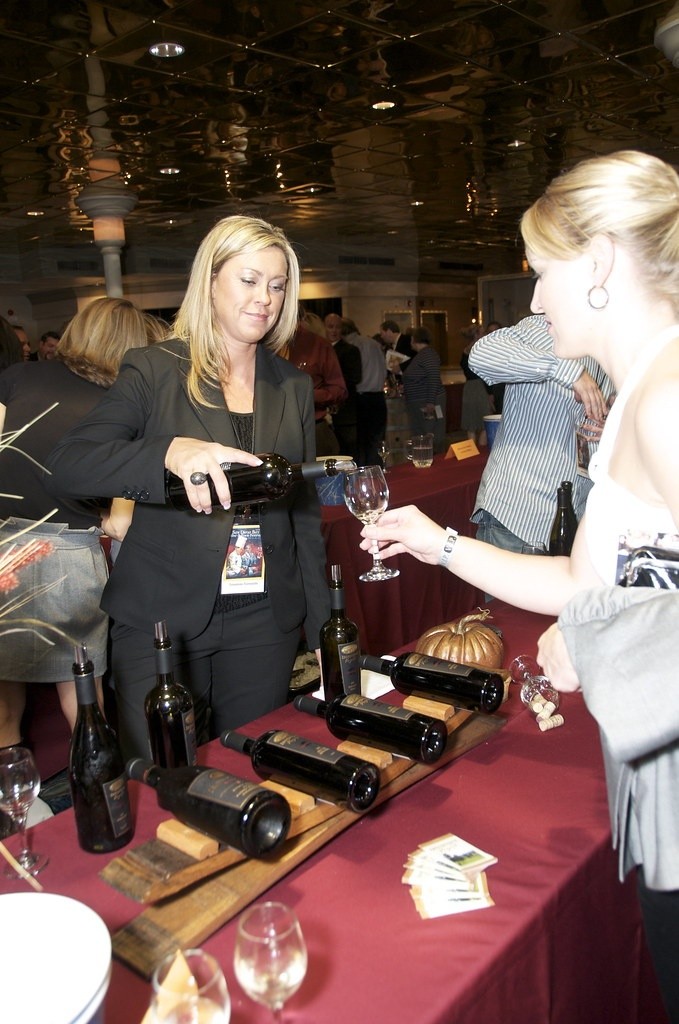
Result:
[414,609,505,670]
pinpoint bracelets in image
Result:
[437,527,458,568]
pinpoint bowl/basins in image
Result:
[1,891,114,1024]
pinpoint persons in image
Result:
[578,440,589,469]
[43,214,334,771]
[226,535,261,578]
[0,313,60,367]
[0,298,167,749]
[467,315,619,603]
[278,314,507,468]
[359,149,679,1024]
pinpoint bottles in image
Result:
[221,728,381,812]
[293,692,448,766]
[561,481,577,537]
[319,564,361,701]
[549,488,574,558]
[165,451,357,507]
[68,645,134,854]
[144,620,198,768]
[126,755,290,858]
[359,650,504,714]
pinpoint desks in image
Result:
[0,441,497,791]
[442,367,467,430]
[0,601,671,1024]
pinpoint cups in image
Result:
[483,414,501,448]
[521,541,547,556]
[152,947,230,1023]
[403,435,434,468]
[314,454,354,505]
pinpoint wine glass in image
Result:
[233,902,308,1024]
[377,440,392,473]
[508,654,560,712]
[342,465,400,581]
[0,747,49,880]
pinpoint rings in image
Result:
[190,472,208,486]
